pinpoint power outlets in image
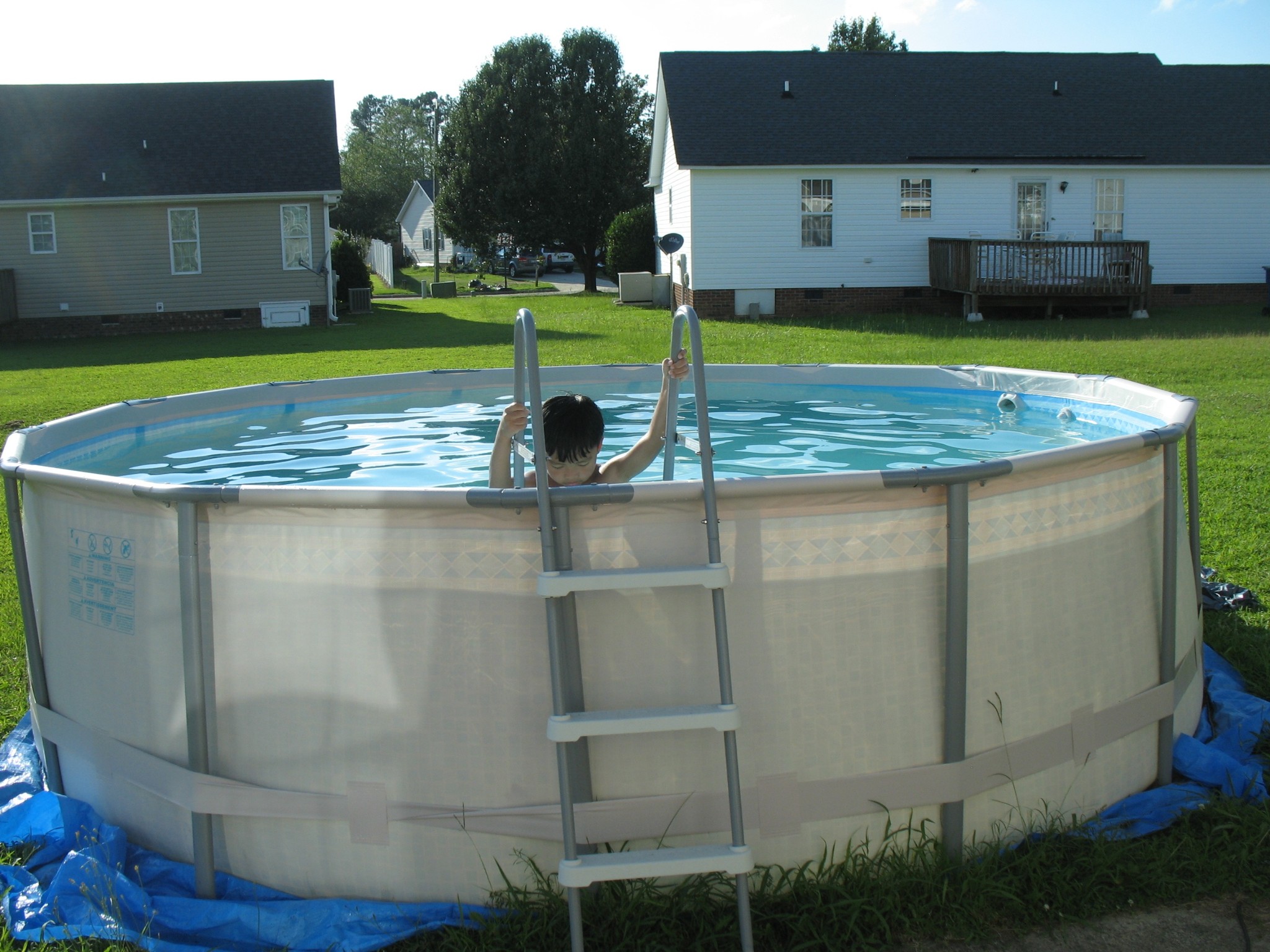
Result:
[157,302,164,313]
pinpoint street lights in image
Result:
[432,97,443,284]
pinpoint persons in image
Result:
[488,347,691,489]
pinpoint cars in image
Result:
[487,246,546,278]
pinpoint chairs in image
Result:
[969,230,1076,283]
[1101,232,1135,284]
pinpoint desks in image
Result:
[995,247,1045,278]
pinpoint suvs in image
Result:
[540,243,575,273]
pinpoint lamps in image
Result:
[1061,181,1069,193]
